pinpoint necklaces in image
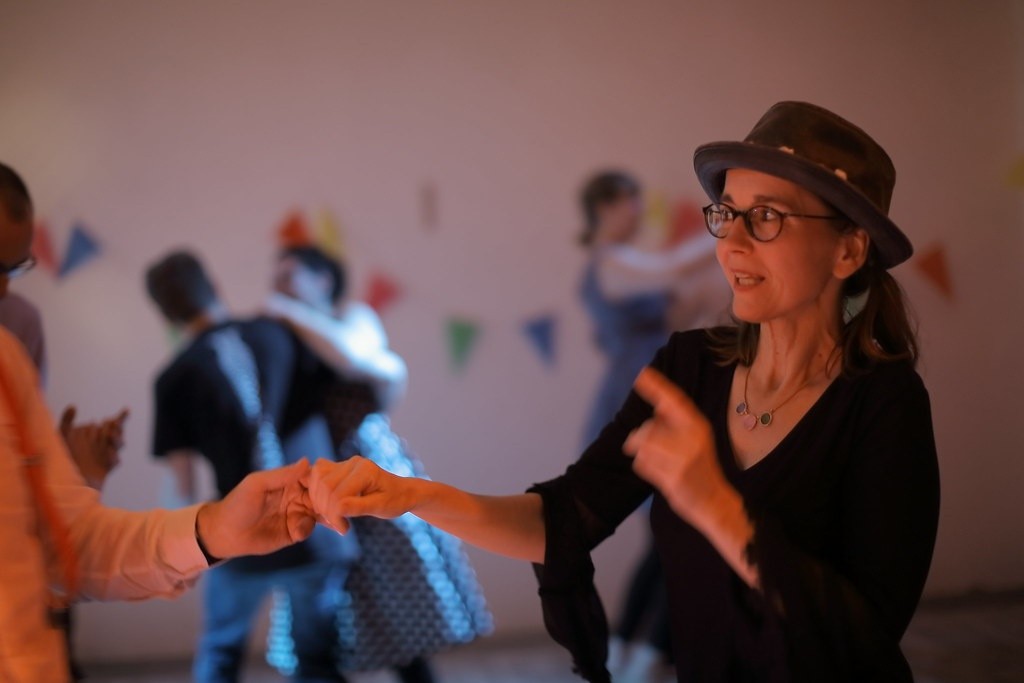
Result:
[735,362,823,430]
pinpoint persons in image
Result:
[312,100,940,683]
[0,159,132,683]
[141,158,718,682]
[0,324,320,683]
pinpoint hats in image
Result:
[692,101,913,269]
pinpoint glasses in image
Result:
[702,202,857,242]
[0,256,38,280]
